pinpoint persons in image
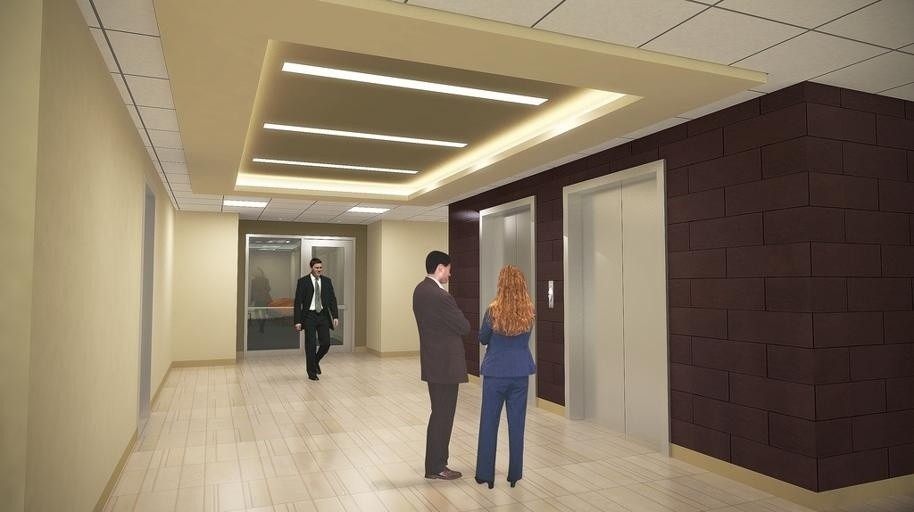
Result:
[474,264,538,489]
[412,250,472,481]
[249,267,273,334]
[294,257,339,381]
